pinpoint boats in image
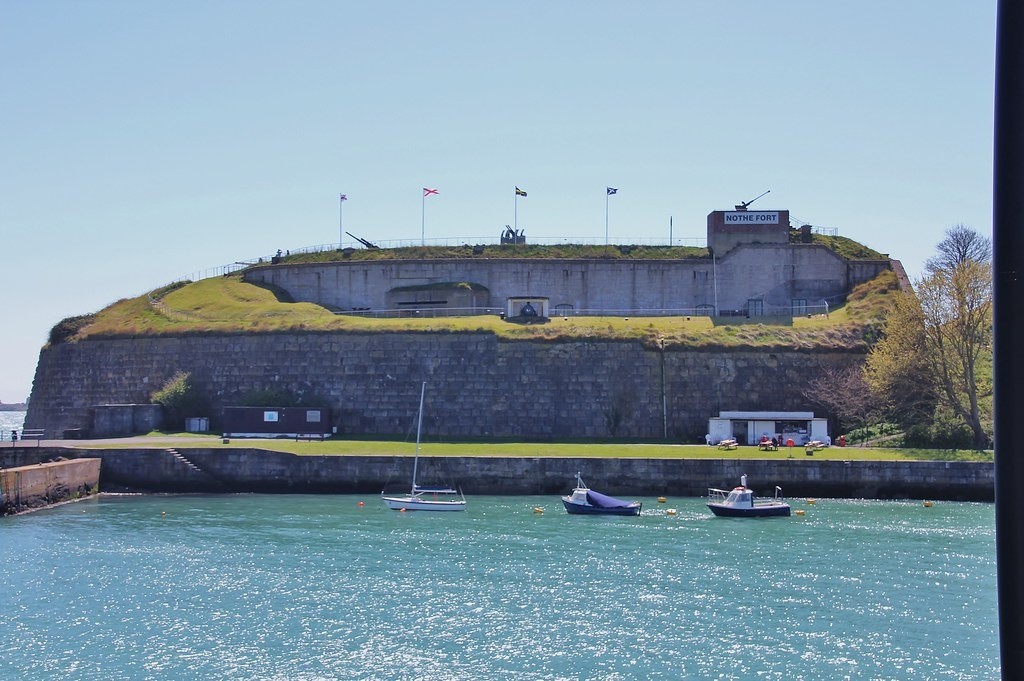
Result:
[705,473,791,518]
[561,470,645,516]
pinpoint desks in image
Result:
[759,441,771,451]
[718,439,735,451]
[805,440,821,451]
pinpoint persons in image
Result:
[771,436,778,447]
[758,435,767,446]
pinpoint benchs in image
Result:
[717,443,739,447]
[296,431,324,442]
[10,428,46,439]
[769,444,779,447]
[804,444,825,448]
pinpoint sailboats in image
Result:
[380,381,469,511]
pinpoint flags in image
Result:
[340,194,348,200]
[606,187,618,195]
[424,187,440,197]
[515,186,527,197]
